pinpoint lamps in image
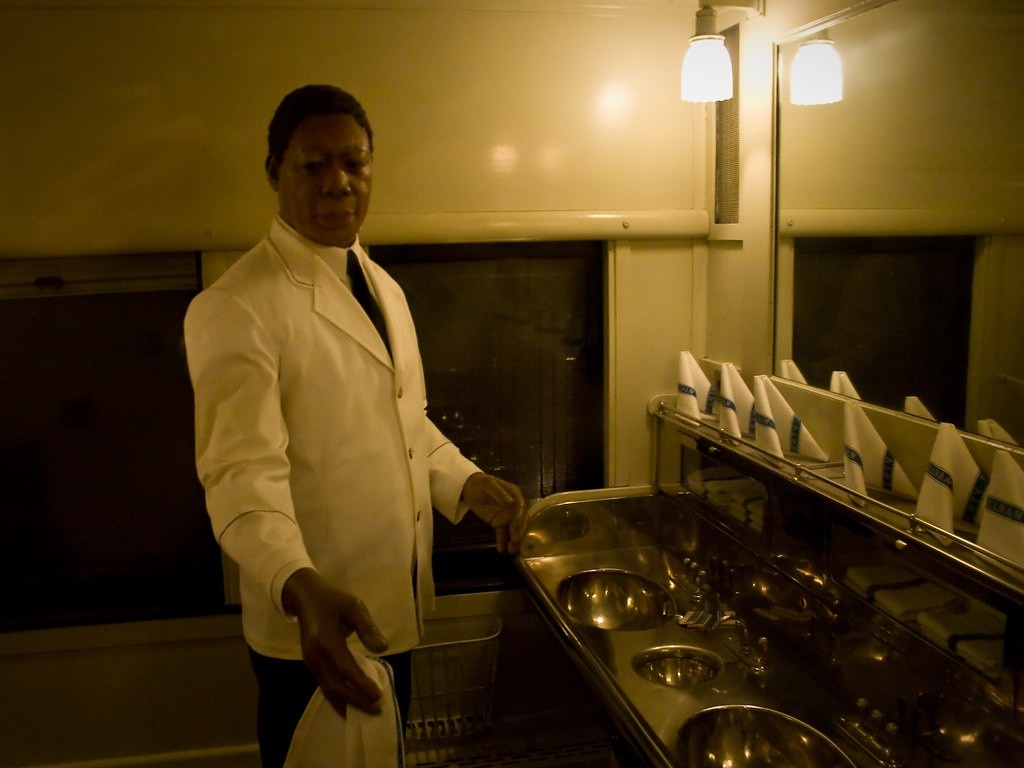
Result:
[790,27,847,106]
[682,1,766,104]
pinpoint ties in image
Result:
[346,249,393,363]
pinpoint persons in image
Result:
[181,81,530,768]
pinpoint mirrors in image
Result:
[761,2,1024,451]
